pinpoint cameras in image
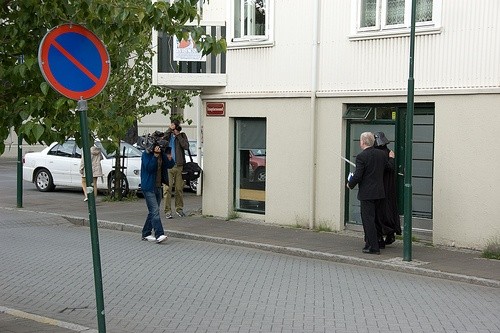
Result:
[173,126,181,135]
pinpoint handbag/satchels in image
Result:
[181,162,201,180]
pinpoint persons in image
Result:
[80,136,103,202]
[162,119,189,218]
[141,131,174,242]
[347,131,402,254]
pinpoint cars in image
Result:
[121,138,204,192]
[247,148,266,181]
[22,137,149,197]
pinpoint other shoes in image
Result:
[84,198,88,201]
[377,238,385,248]
[361,244,380,253]
[385,234,396,245]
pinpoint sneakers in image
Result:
[176,210,185,218]
[157,235,167,243]
[141,235,157,241]
[166,213,172,218]
